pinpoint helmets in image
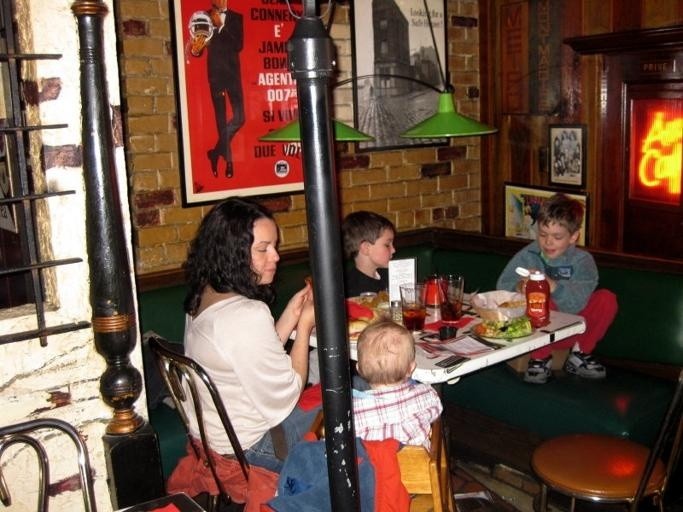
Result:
[188,10,214,42]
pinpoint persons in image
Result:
[490,192,618,386]
[336,208,398,299]
[188,0,245,179]
[551,129,580,178]
[171,194,370,478]
[348,320,443,452]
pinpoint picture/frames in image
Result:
[166,0,311,210]
[346,0,452,154]
[501,179,591,248]
[546,121,588,192]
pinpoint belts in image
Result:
[220,425,288,461]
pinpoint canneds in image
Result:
[360,292,377,303]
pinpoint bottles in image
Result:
[390,299,401,327]
[515,267,548,328]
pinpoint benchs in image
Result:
[134,225,436,483]
[433,223,683,452]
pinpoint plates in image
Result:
[479,331,534,343]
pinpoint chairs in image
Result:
[282,285,587,473]
[0,418,98,512]
[525,366,682,512]
[305,406,457,511]
[140,329,253,511]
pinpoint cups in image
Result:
[359,293,379,310]
[399,281,426,336]
[438,274,462,322]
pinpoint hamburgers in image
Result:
[349,320,370,340]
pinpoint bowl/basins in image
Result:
[471,289,527,324]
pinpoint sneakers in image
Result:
[563,352,607,378]
[524,356,553,384]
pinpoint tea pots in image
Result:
[423,274,448,329]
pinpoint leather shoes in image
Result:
[226,159,232,176]
[207,150,219,176]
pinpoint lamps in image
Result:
[255,0,500,144]
